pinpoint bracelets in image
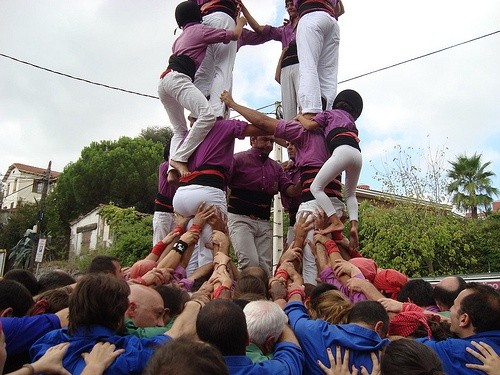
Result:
[276,269,289,280]
[325,240,340,255]
[287,290,303,299]
[176,227,184,235]
[24,364,34,375]
[172,240,188,255]
[190,224,202,234]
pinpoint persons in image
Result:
[293,0,345,120]
[294,89,364,251]
[0,200,500,375]
[158,0,248,186]
[173,0,344,282]
[152,145,176,248]
